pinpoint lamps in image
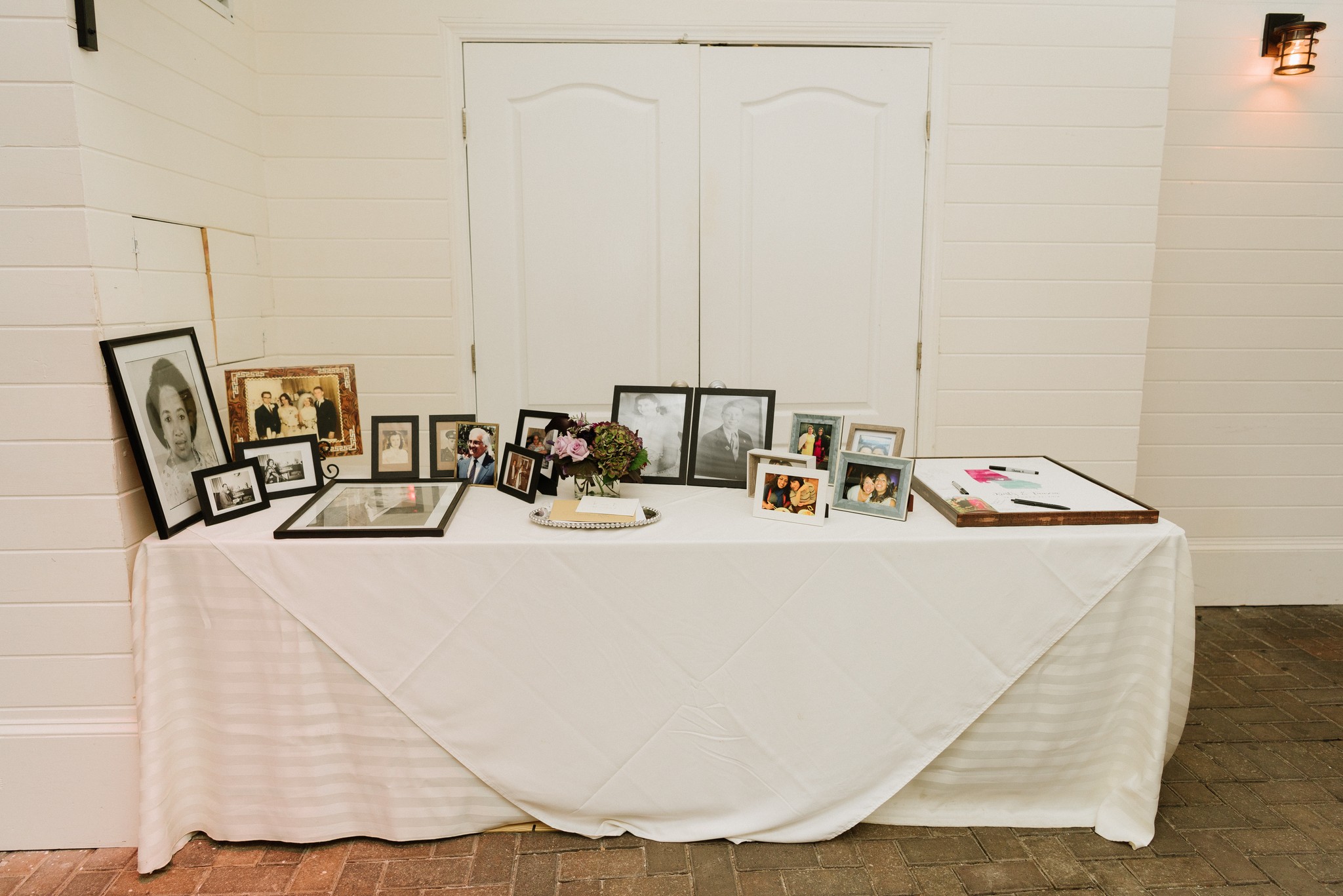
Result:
[1263,13,1326,77]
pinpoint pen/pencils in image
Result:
[952,481,969,495]
[989,465,1039,475]
[1011,499,1071,510]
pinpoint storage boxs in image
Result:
[900,456,1160,528]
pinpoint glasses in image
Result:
[467,440,483,446]
[262,397,272,399]
[818,431,823,432]
[314,392,320,394]
[778,477,789,484]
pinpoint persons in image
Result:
[457,447,465,470]
[872,445,888,456]
[890,481,899,499]
[145,357,220,509]
[219,484,238,509]
[382,431,408,464]
[528,434,548,469]
[694,401,755,481]
[456,427,495,486]
[534,441,545,452]
[813,428,829,469]
[299,397,320,442]
[305,487,436,526]
[798,425,815,456]
[265,459,288,484]
[277,393,299,437]
[514,464,524,480]
[313,386,336,440]
[254,391,281,441]
[845,463,863,488]
[441,430,456,462]
[789,476,816,506]
[769,459,781,465]
[762,474,791,510]
[858,445,873,454]
[462,448,471,460]
[631,393,682,478]
[868,471,896,507]
[847,473,877,503]
[780,461,792,466]
[506,459,517,486]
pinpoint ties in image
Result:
[317,401,319,407]
[512,467,514,479]
[468,459,478,484]
[268,405,273,414]
[731,432,738,462]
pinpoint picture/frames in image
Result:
[752,463,830,527]
[846,423,905,457]
[371,415,419,479]
[610,385,694,485]
[223,363,363,457]
[273,478,470,538]
[830,450,916,521]
[686,388,776,489]
[454,421,500,488]
[234,434,324,501]
[429,414,476,477]
[97,327,231,540]
[190,456,271,526]
[496,442,544,504]
[786,411,845,487]
[746,448,816,500]
[373,483,460,515]
[514,409,569,496]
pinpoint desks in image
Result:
[131,483,1196,871]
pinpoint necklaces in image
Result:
[170,449,201,495]
[819,438,821,439]
[861,490,871,499]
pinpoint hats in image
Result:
[445,430,456,440]
